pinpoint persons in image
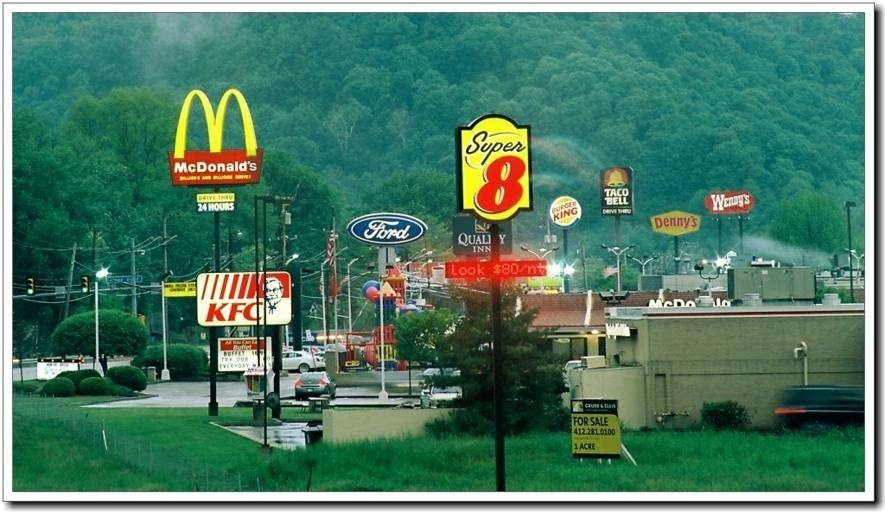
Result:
[261,278,290,324]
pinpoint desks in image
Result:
[308,397,329,413]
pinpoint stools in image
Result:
[328,405,336,409]
[300,406,309,412]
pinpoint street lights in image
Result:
[398,249,433,283]
[130,249,146,316]
[845,201,857,303]
[228,231,242,272]
[283,254,298,274]
[159,269,174,380]
[601,243,658,291]
[95,267,109,379]
[519,245,559,260]
[347,255,364,332]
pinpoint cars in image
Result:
[281,345,326,373]
[295,371,335,401]
[418,368,463,409]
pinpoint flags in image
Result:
[324,220,335,268]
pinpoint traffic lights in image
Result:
[81,275,90,295]
[25,276,35,296]
